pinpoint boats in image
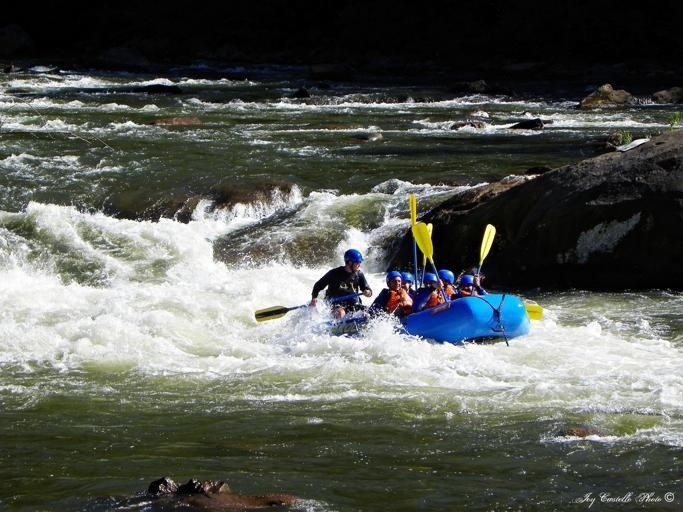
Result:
[300,293,530,345]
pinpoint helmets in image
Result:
[344,249,363,264]
[386,269,475,288]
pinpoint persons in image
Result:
[310,248,373,320]
[370,267,488,318]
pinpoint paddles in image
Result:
[420,223,433,287]
[471,224,496,296]
[525,303,543,320]
[409,193,417,293]
[255,293,364,322]
[412,221,449,304]
